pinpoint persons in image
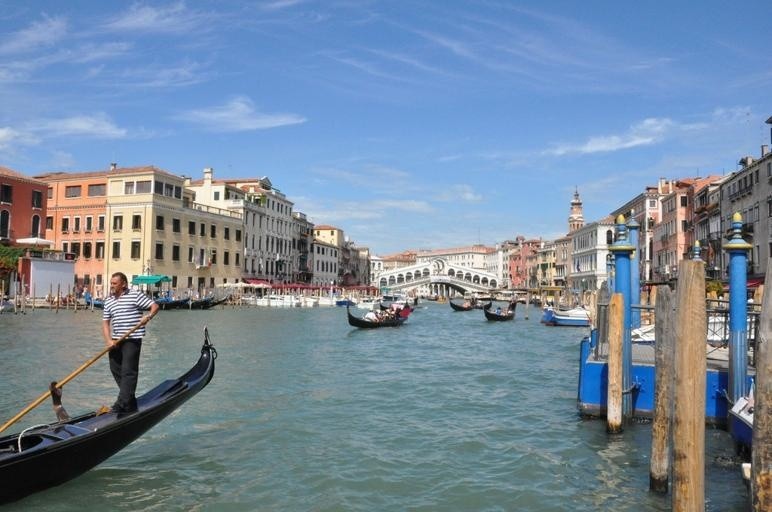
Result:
[470,290,518,315]
[366,302,405,320]
[99,271,159,418]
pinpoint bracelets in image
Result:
[149,314,154,317]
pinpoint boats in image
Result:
[242,292,358,308]
[129,274,228,309]
[539,301,591,328]
[628,316,756,346]
[344,289,528,330]
[0,322,220,504]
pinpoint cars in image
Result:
[3,295,10,301]
